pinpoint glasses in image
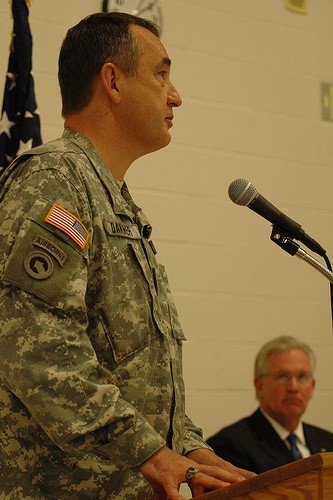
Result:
[262,371,312,385]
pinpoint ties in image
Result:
[286,433,302,460]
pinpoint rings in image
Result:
[185,467,199,489]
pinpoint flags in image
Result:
[0,0,43,174]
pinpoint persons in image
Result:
[202,335,333,476]
[0,12,260,500]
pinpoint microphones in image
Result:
[228,178,327,256]
